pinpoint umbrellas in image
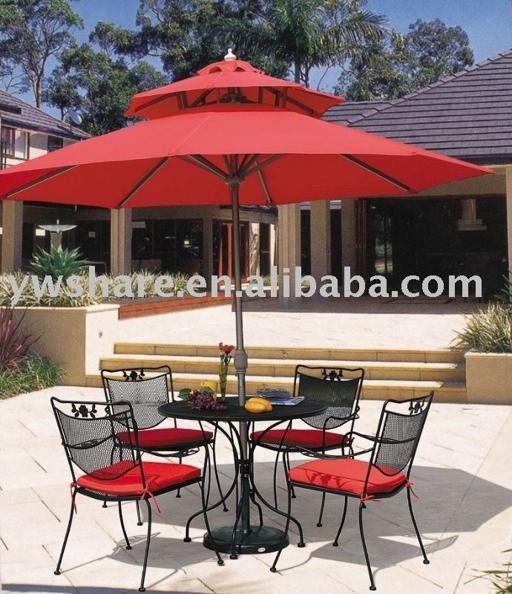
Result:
[0,48,496,530]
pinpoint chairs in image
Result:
[50,396,224,591]
[270,390,433,591]
[250,365,364,528]
[101,365,228,526]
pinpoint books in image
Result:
[266,396,305,405]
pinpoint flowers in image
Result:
[218,342,235,362]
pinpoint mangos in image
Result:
[244,398,272,413]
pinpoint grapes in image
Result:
[187,389,227,411]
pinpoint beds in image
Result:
[157,395,326,552]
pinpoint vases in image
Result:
[216,365,229,403]
